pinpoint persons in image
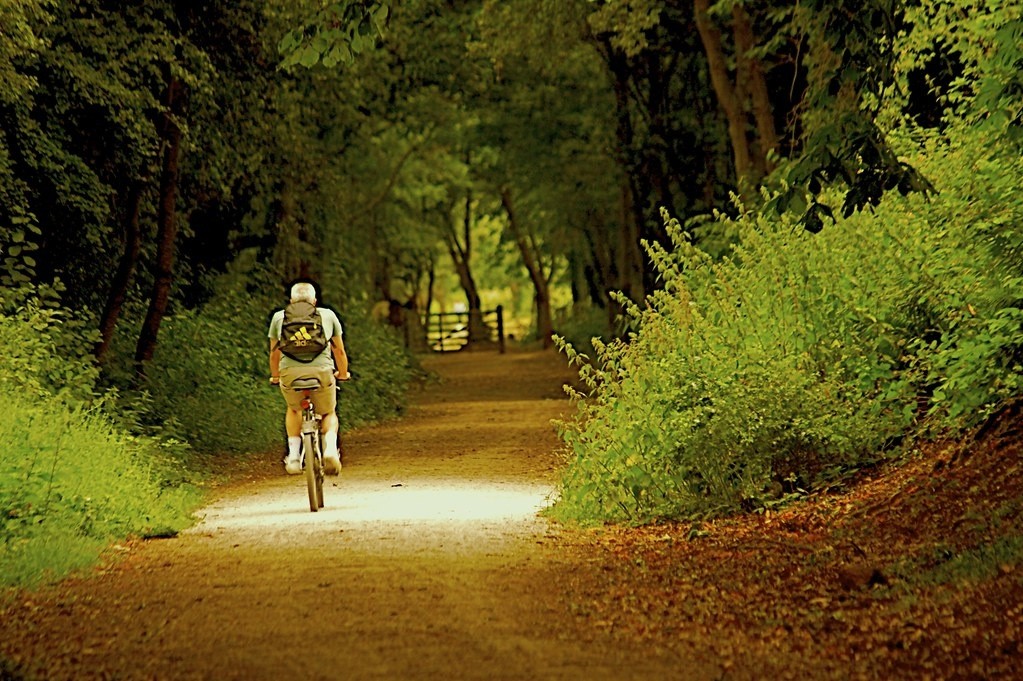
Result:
[267,282,350,476]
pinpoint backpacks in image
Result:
[272,301,337,363]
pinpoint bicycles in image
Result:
[267,372,352,513]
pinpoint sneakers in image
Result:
[323,448,342,476]
[284,453,303,475]
